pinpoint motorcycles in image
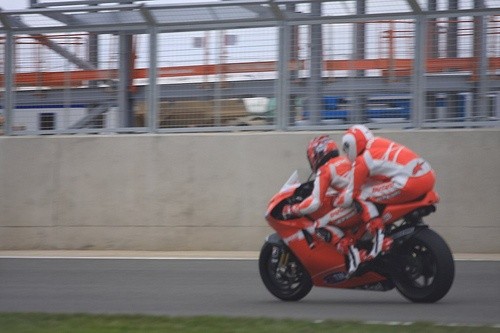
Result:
[258,169,456,304]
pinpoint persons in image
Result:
[329,123,435,262]
[282,134,386,278]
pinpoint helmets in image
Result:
[306,134,339,173]
[341,124,373,161]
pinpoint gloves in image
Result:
[281,203,302,220]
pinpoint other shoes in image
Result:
[346,245,368,277]
[367,230,394,261]
